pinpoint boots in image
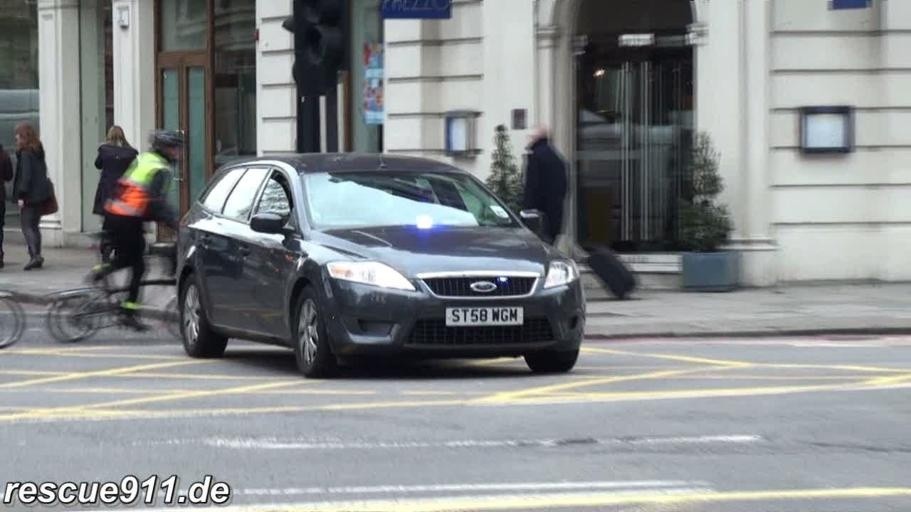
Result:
[24,239,43,269]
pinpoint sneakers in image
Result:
[118,312,147,330]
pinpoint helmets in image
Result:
[153,132,182,145]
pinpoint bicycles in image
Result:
[47,231,183,342]
[1,290,26,349]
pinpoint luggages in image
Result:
[587,246,634,297]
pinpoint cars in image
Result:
[580,105,691,148]
[174,151,582,376]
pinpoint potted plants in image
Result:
[669,127,741,294]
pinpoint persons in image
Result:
[11,123,48,271]
[0,144,14,269]
[90,128,186,332]
[91,124,139,262]
[521,125,568,247]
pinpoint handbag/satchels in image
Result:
[41,181,58,215]
